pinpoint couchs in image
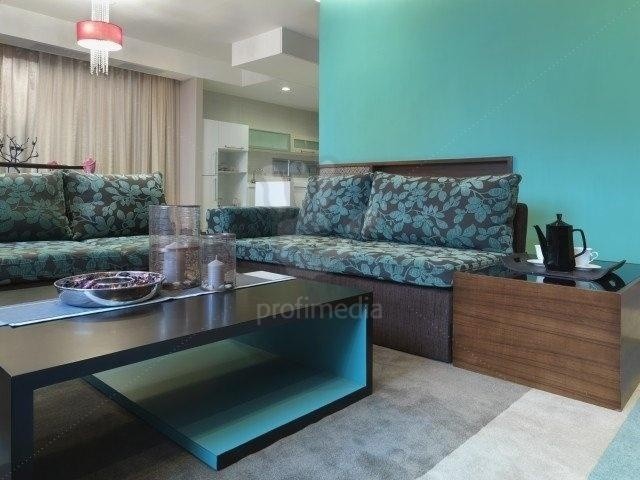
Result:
[205,202,528,363]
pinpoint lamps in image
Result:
[76,0,123,76]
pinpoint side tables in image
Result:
[452,255,640,412]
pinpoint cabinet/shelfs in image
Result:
[200,119,249,232]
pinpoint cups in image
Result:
[534,245,544,260]
[574,247,598,267]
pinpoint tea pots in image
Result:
[533,214,586,273]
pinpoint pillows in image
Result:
[63,165,175,241]
[361,170,522,253]
[296,172,374,239]
[0,169,73,243]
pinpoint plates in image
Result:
[54,270,165,307]
[527,259,602,272]
